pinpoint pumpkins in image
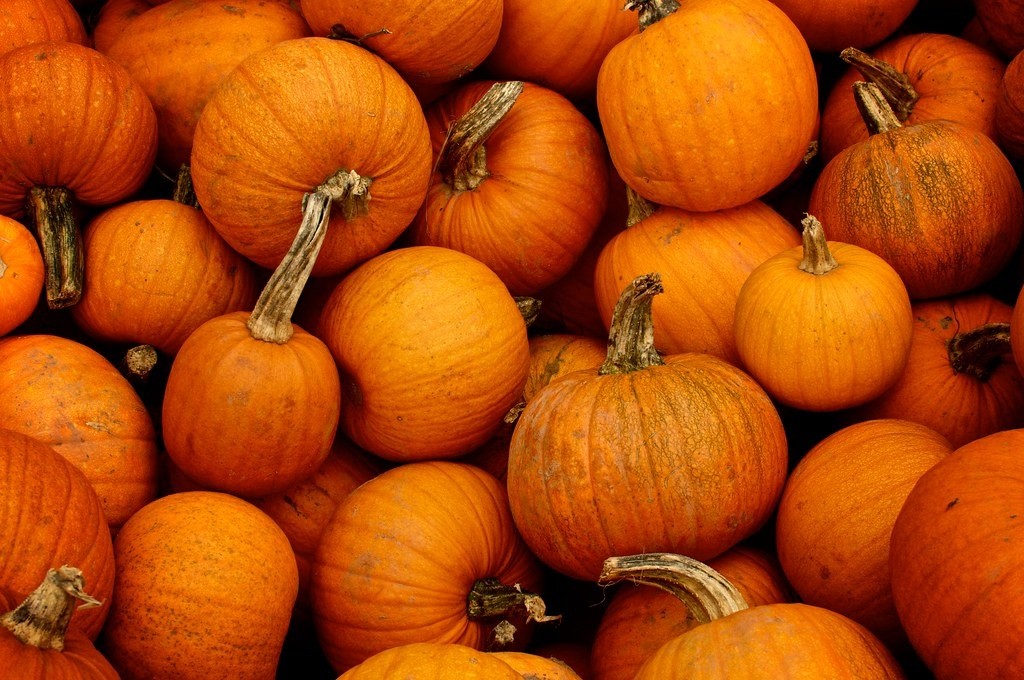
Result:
[0,0,1024,680]
